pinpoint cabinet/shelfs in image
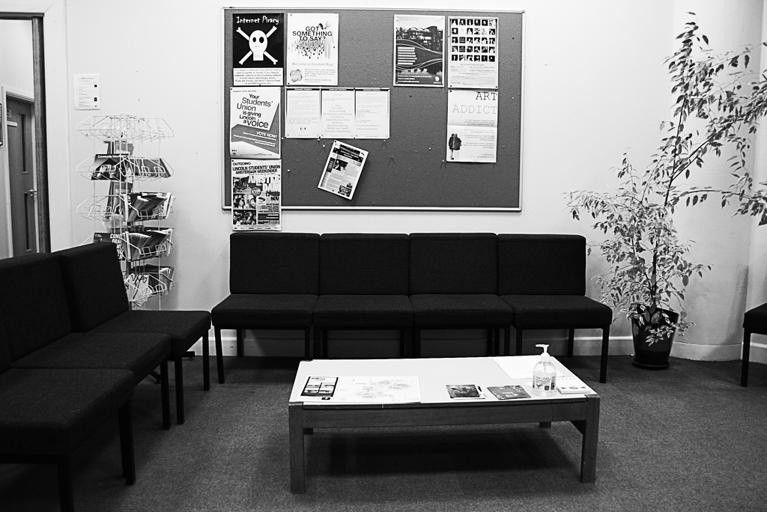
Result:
[75,115,195,384]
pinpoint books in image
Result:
[445,384,484,399]
[486,385,531,400]
[90,141,174,295]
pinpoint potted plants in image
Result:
[561,11,766,370]
